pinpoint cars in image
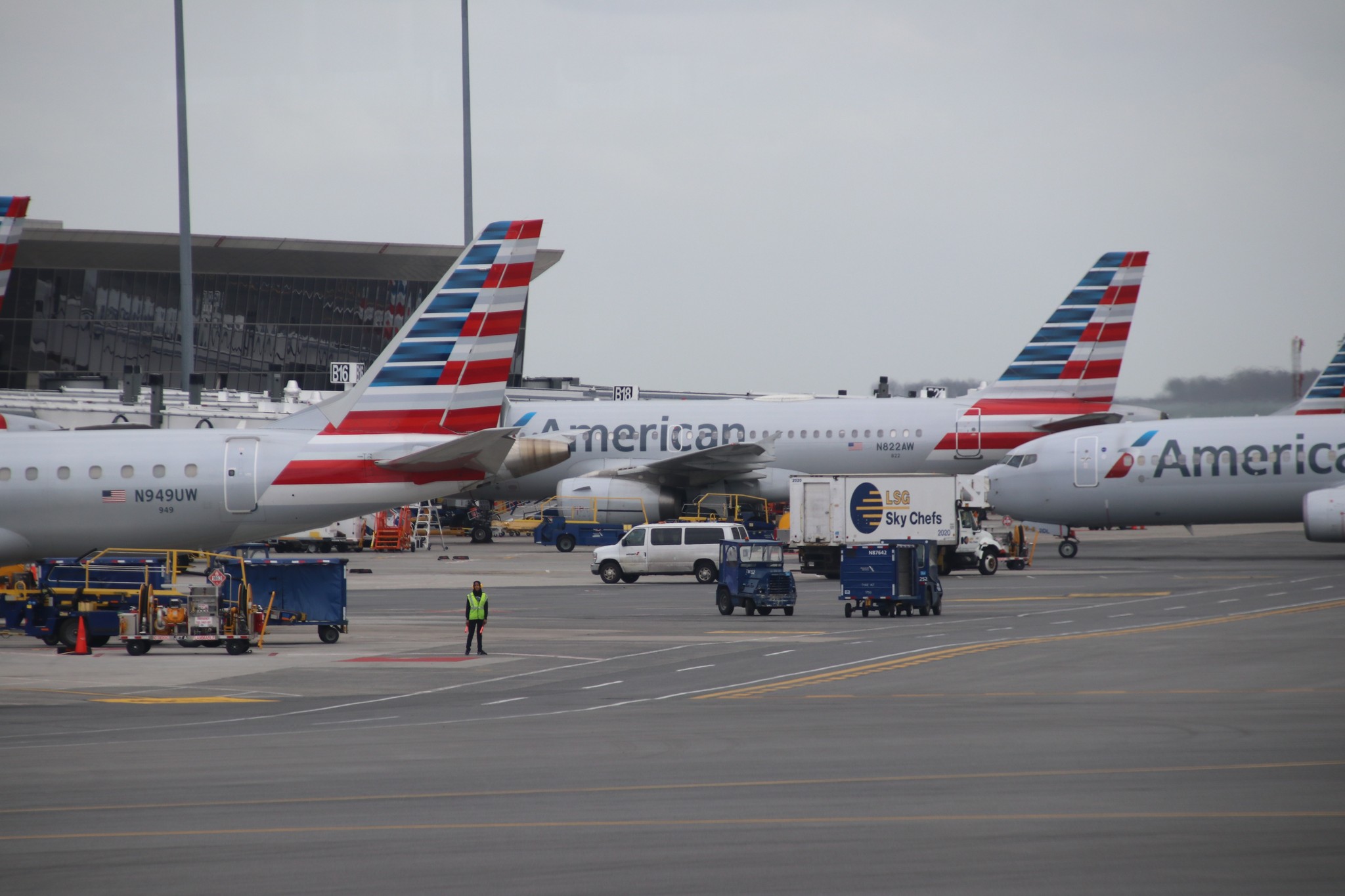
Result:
[715,539,798,616]
[837,542,944,617]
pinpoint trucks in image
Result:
[786,471,1006,577]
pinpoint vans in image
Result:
[589,521,751,584]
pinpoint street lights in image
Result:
[72,616,89,655]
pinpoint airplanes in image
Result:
[0,215,573,574]
[969,327,1345,556]
[498,247,1169,529]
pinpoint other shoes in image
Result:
[465,648,471,655]
[477,650,487,655]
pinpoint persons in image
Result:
[464,580,488,655]
